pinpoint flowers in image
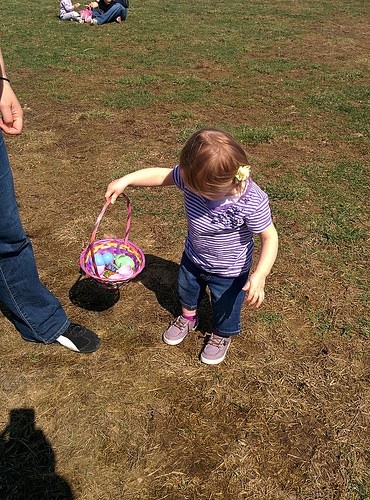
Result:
[235,165,251,184]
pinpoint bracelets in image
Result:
[0,76,10,83]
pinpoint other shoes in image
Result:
[91,19,97,26]
[78,20,84,24]
[116,16,121,23]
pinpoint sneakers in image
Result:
[22,322,102,353]
[200,332,231,365]
[163,316,198,345]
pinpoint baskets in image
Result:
[79,192,146,290]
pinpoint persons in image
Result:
[0,46,103,354]
[59,0,84,24]
[87,0,129,26]
[103,127,279,365]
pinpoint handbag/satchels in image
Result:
[79,5,92,22]
